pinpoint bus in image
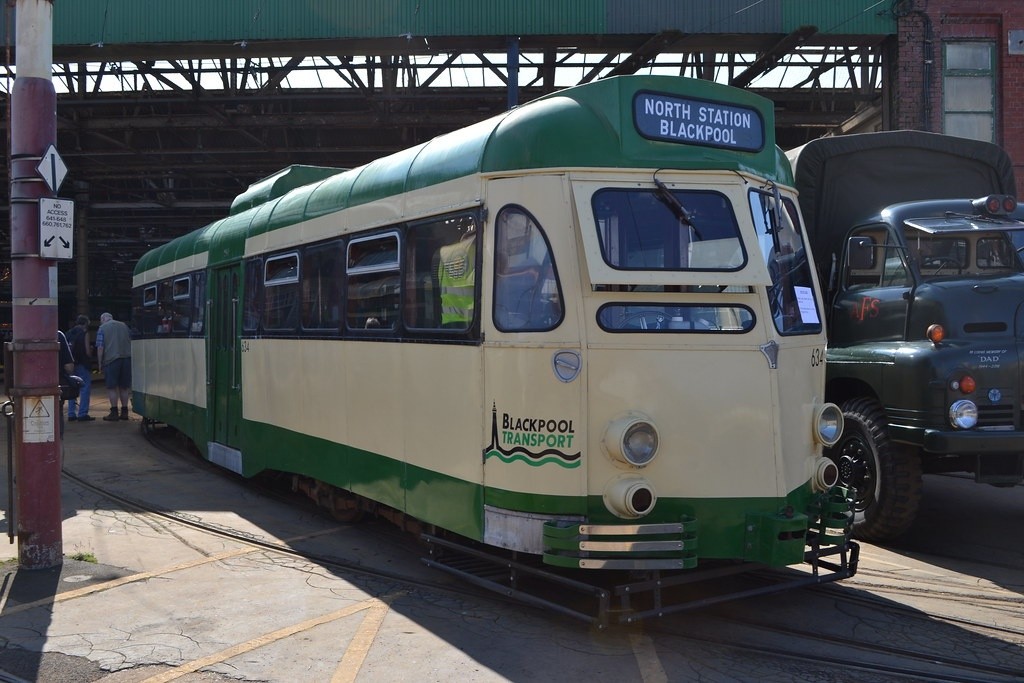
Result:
[131,76,860,626]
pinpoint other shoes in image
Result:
[103,408,119,421]
[77,415,96,421]
[119,407,129,420]
[68,416,77,421]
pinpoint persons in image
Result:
[57,331,75,439]
[65,315,96,421]
[95,313,132,421]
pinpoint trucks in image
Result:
[781,130,1024,539]
